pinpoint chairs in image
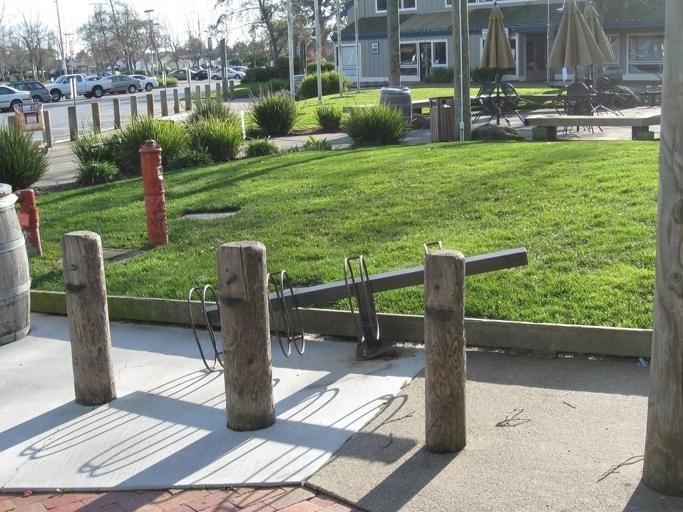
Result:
[550,76,629,129]
[468,82,534,127]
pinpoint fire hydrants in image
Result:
[15,189,42,258]
[138,139,169,248]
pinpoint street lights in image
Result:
[64,33,74,75]
[145,9,160,76]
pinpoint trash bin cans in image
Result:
[429,96,455,143]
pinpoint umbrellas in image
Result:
[479,0,516,123]
[581,2,615,105]
[546,0,607,131]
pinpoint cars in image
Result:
[167,64,250,80]
[0,79,55,112]
[102,73,160,95]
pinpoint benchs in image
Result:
[523,114,660,140]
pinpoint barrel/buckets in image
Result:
[381,85,412,132]
[1,190,32,344]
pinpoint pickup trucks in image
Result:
[44,72,113,102]
